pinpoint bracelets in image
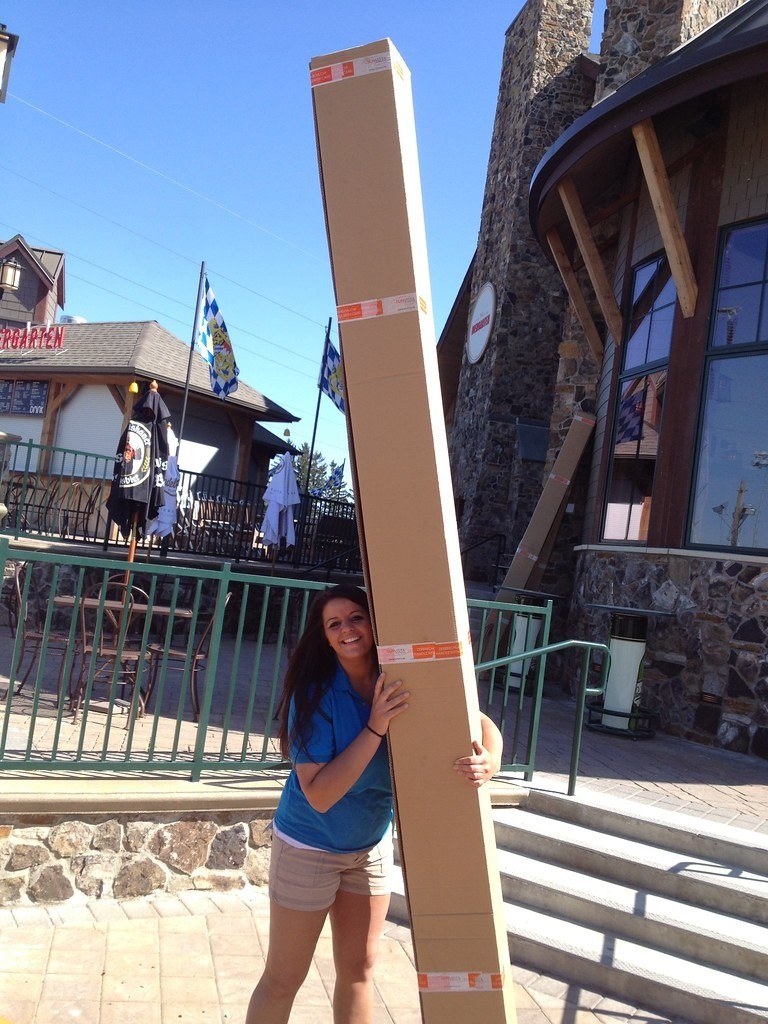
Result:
[366,724,385,739]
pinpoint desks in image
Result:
[1,480,57,528]
[584,603,677,742]
[46,594,194,717]
[492,584,566,693]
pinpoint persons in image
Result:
[246,583,504,1024]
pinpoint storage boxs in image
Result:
[308,38,598,1024]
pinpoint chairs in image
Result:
[3,473,270,724]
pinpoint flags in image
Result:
[316,336,346,415]
[192,272,240,401]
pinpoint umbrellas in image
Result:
[260,453,300,564]
[105,380,171,700]
[150,454,181,553]
